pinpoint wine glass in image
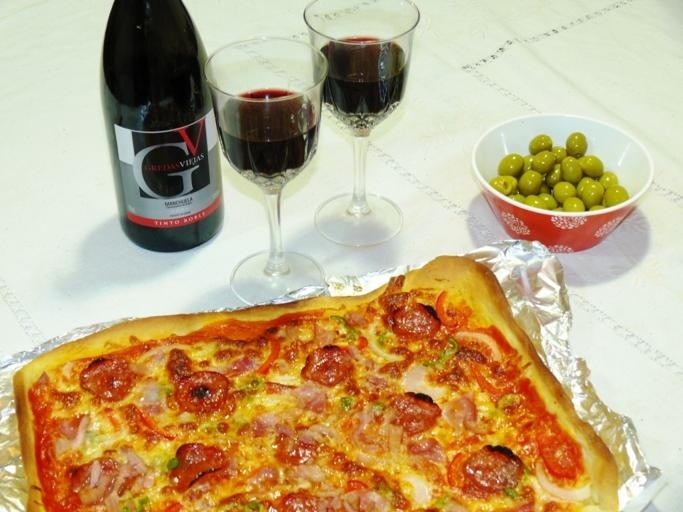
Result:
[301,0,420,250]
[201,34,331,300]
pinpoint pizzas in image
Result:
[15,256,618,512]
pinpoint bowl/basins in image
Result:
[473,113,656,251]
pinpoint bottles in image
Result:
[98,1,224,254]
